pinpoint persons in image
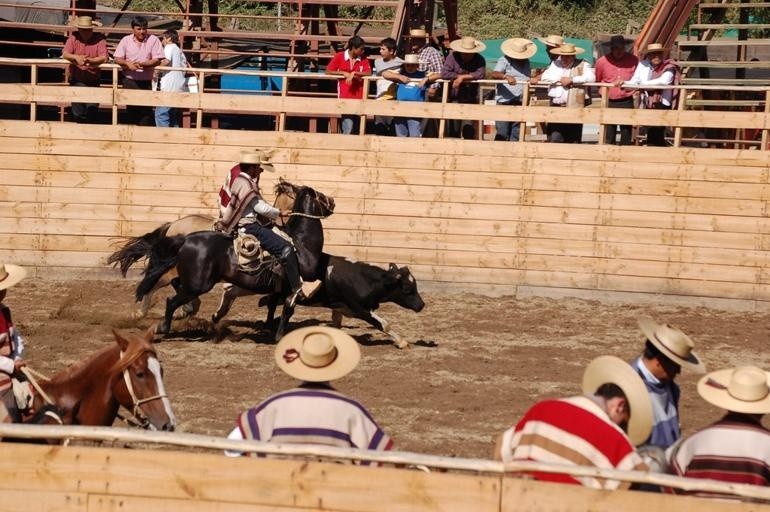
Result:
[628,315,707,452]
[493,355,655,494]
[218,149,273,220]
[61,16,107,123]
[217,151,322,301]
[113,15,166,126]
[0,260,27,424]
[659,366,770,505]
[153,29,187,127]
[223,325,398,468]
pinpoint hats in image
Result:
[401,29,434,39]
[500,37,538,60]
[639,43,667,53]
[696,365,770,416]
[538,34,564,48]
[636,315,706,374]
[601,36,633,46]
[0,262,27,291]
[580,354,654,448]
[449,36,486,54]
[273,325,362,384]
[72,15,103,30]
[396,53,431,65]
[548,43,585,56]
[237,146,273,168]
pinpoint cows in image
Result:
[211,251,425,351]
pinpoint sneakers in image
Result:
[292,278,322,303]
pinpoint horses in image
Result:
[133,184,336,341]
[106,176,300,324]
[0,323,177,447]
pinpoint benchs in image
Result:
[677,1,770,112]
